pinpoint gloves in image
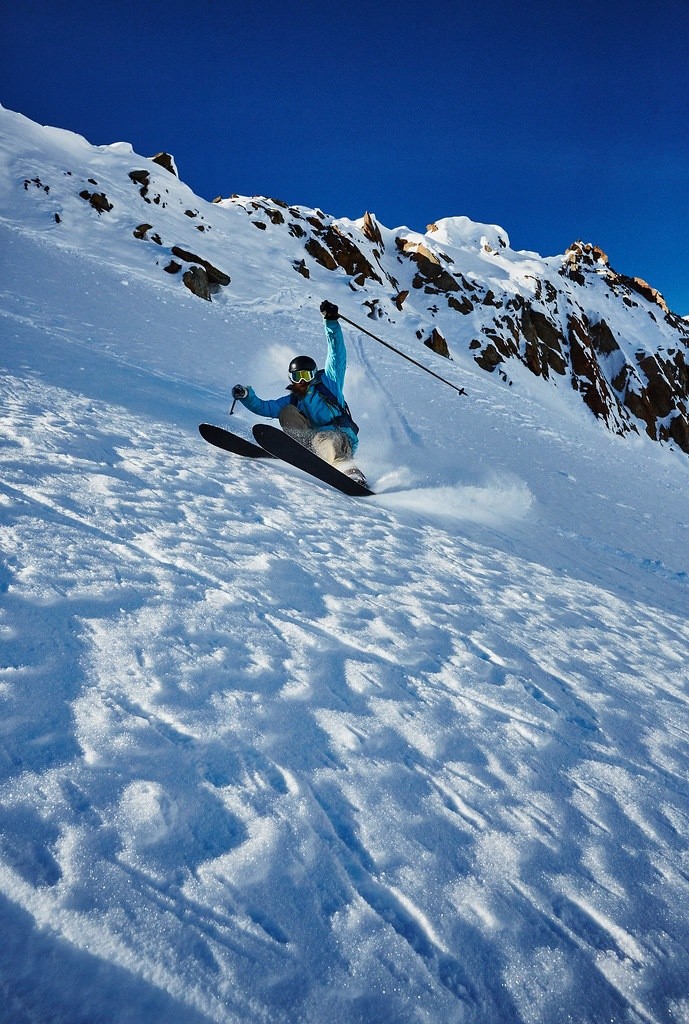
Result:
[320,300,339,320]
[232,384,245,400]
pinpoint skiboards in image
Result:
[198,423,375,496]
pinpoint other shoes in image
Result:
[354,479,367,487]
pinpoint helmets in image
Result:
[289,356,316,372]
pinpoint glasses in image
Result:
[288,372,314,383]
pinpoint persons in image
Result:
[231,299,368,490]
